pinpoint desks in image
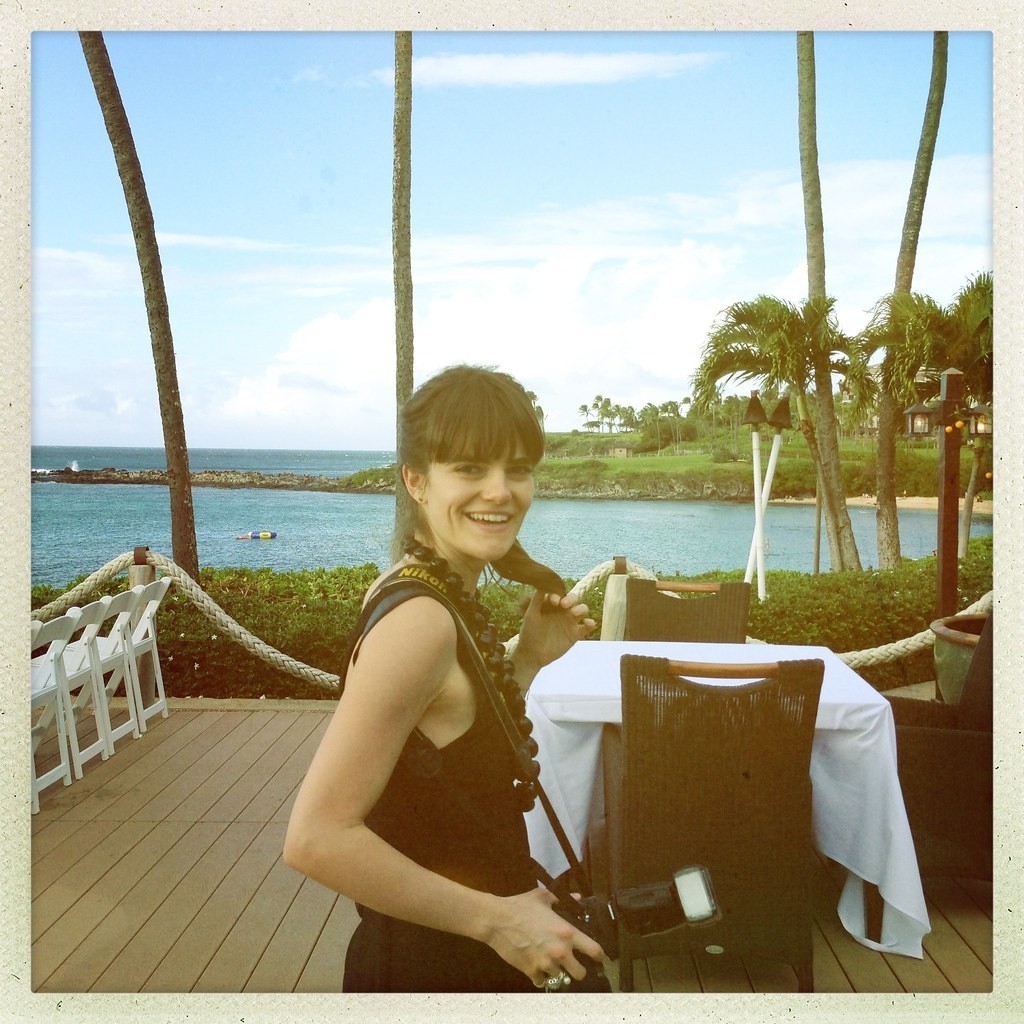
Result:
[525,639,929,958]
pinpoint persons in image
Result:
[282,367,614,992]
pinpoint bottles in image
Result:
[485,870,714,976]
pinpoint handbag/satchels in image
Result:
[385,901,614,993]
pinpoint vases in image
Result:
[928,614,986,704]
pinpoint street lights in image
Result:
[900,368,992,704]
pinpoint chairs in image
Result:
[882,610,994,885]
[622,576,751,645]
[29,575,171,816]
[582,654,826,993]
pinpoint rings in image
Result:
[534,972,570,989]
